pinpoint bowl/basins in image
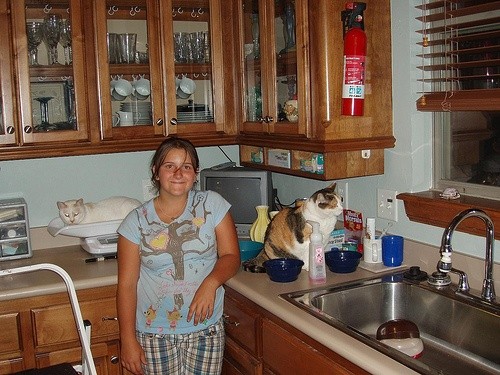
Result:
[138,52,150,63]
[239,241,264,263]
[263,257,305,283]
[325,251,362,273]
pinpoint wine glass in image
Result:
[26,13,72,67]
[33,96,57,132]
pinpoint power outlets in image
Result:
[334,181,348,210]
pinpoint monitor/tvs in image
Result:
[200,168,274,239]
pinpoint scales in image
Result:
[47,217,123,254]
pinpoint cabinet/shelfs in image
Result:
[0,0,94,161]
[222,284,373,375]
[94,0,225,154]
[222,0,396,179]
[0,198,32,260]
[0,282,123,375]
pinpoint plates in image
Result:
[121,102,152,125]
[177,111,213,123]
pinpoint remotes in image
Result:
[211,162,236,171]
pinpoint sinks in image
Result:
[277,268,500,375]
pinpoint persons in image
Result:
[116,137,241,375]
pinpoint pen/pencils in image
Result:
[85,254,117,263]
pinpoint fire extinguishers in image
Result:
[342,3,369,117]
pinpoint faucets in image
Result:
[438,208,500,310]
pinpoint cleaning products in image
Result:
[366,217,376,240]
[306,220,327,284]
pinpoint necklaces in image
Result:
[157,199,187,220]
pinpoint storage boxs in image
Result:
[268,149,290,168]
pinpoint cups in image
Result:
[116,111,141,126]
[175,76,196,100]
[106,31,138,64]
[174,31,210,63]
[381,235,404,267]
[364,235,382,264]
[110,74,150,102]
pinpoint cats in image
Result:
[243,182,344,273]
[52,195,143,236]
